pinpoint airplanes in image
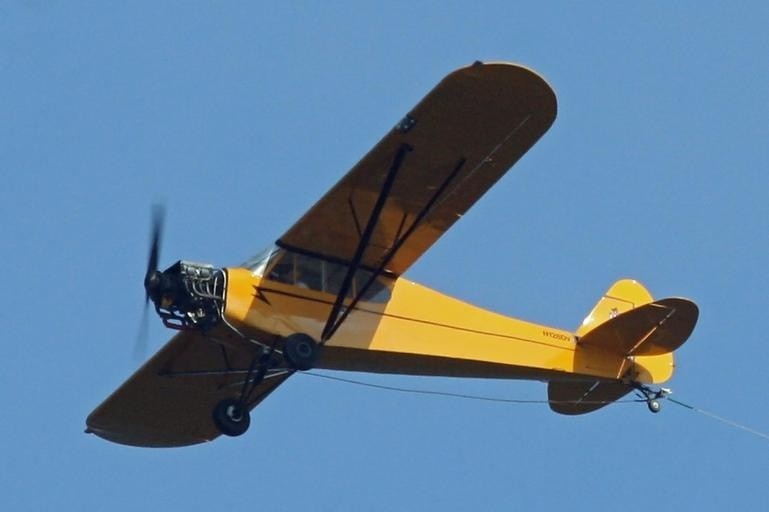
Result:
[79,58,702,449]
